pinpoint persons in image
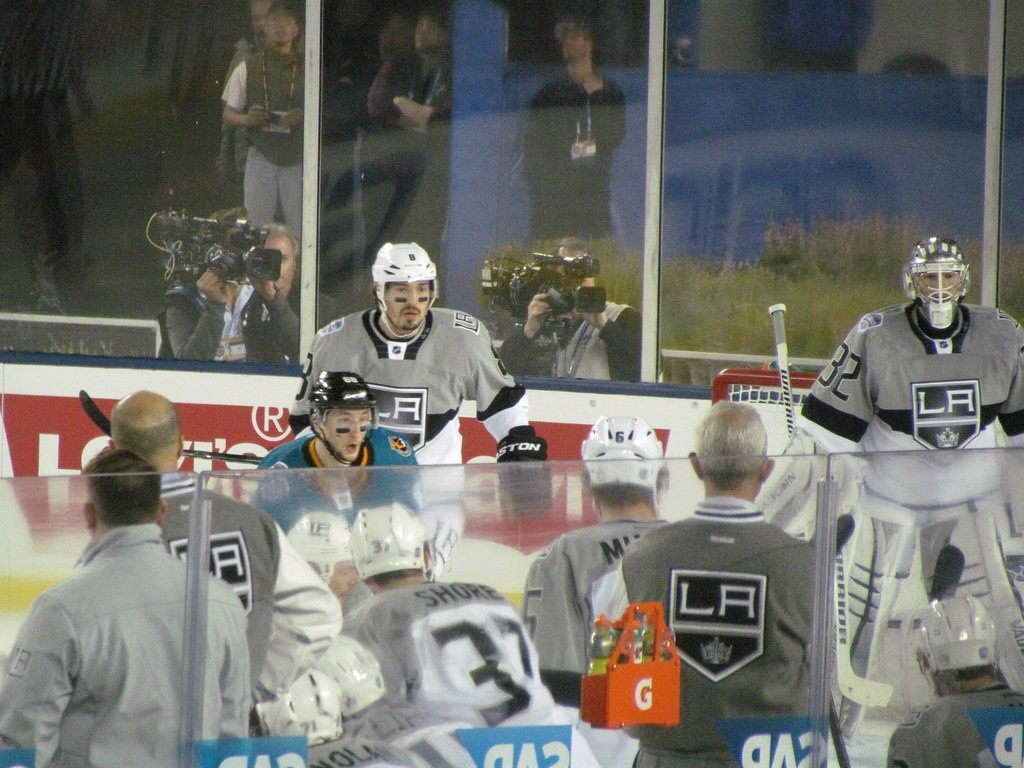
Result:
[596,398,840,768]
[313,630,491,768]
[110,390,344,726]
[754,237,1024,746]
[254,370,465,537]
[499,236,642,382]
[0,450,252,768]
[216,0,625,264]
[285,512,360,599]
[0,0,91,314]
[260,224,300,319]
[521,416,672,707]
[762,0,874,73]
[289,240,553,580]
[255,668,417,768]
[341,502,601,768]
[165,208,300,365]
[630,0,702,69]
[886,594,1024,768]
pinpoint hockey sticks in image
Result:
[766,301,818,541]
[75,387,264,466]
[834,550,895,712]
[929,545,1013,693]
[812,512,856,768]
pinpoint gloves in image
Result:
[497,433,552,512]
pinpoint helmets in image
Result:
[903,237,972,329]
[310,636,387,718]
[286,511,353,565]
[349,502,426,579]
[256,668,343,746]
[371,242,438,308]
[916,596,996,672]
[580,418,663,492]
[308,370,376,421]
